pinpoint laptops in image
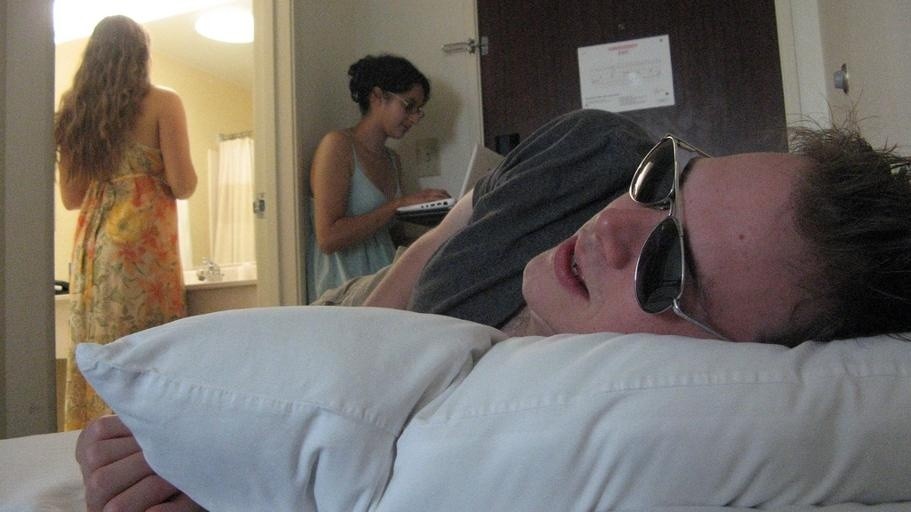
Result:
[395,143,504,212]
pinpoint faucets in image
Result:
[196,257,225,281]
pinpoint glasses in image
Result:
[628,131,735,342]
[379,88,427,121]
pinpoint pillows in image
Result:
[72,301,911,509]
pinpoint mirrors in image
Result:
[53,13,255,272]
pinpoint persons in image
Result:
[52,16,199,432]
[74,105,911,510]
[307,53,453,301]
